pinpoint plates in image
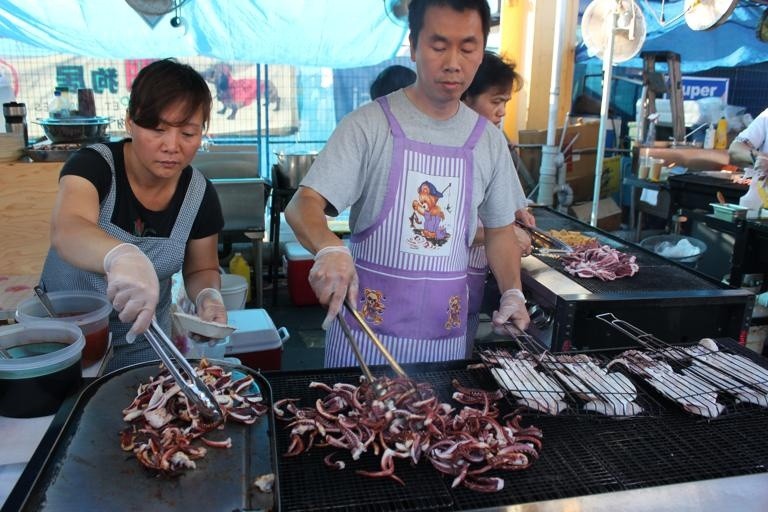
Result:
[0,133,22,161]
[171,311,237,341]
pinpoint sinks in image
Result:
[635,145,676,149]
[205,174,263,184]
[692,145,730,150]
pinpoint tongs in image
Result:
[513,219,577,254]
[330,290,424,414]
[141,319,225,423]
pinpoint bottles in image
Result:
[704,116,728,150]
[47,86,71,118]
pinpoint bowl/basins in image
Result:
[32,116,113,144]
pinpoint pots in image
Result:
[272,150,319,190]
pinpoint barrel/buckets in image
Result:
[221,274,248,312]
[228,253,251,303]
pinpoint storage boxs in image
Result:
[223,307,290,370]
[281,239,352,307]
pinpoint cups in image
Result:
[3,100,29,151]
[638,156,664,180]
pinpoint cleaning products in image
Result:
[715,117,727,149]
[703,122,715,148]
[645,113,660,144]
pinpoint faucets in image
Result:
[684,122,708,140]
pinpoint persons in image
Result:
[462,56,537,359]
[369,65,417,100]
[283,1,530,368]
[34,57,228,380]
[728,107,768,347]
